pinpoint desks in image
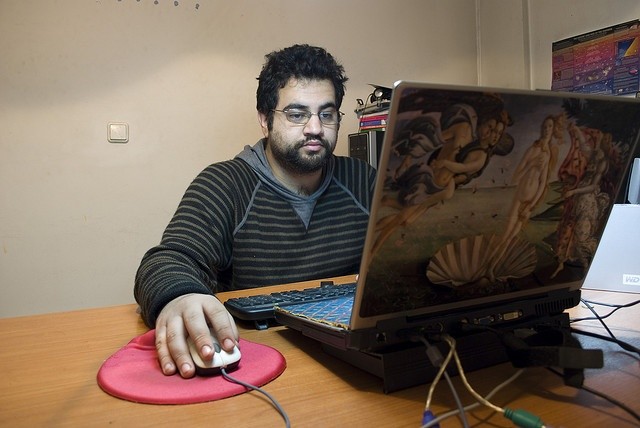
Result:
[0,275,640,428]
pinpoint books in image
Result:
[355,100,390,134]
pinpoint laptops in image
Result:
[273,80,640,385]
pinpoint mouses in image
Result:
[185,327,242,375]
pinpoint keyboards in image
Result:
[223,280,356,330]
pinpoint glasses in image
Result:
[268,105,346,126]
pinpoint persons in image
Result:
[365,90,506,274]
[466,112,608,285]
[132,43,378,380]
